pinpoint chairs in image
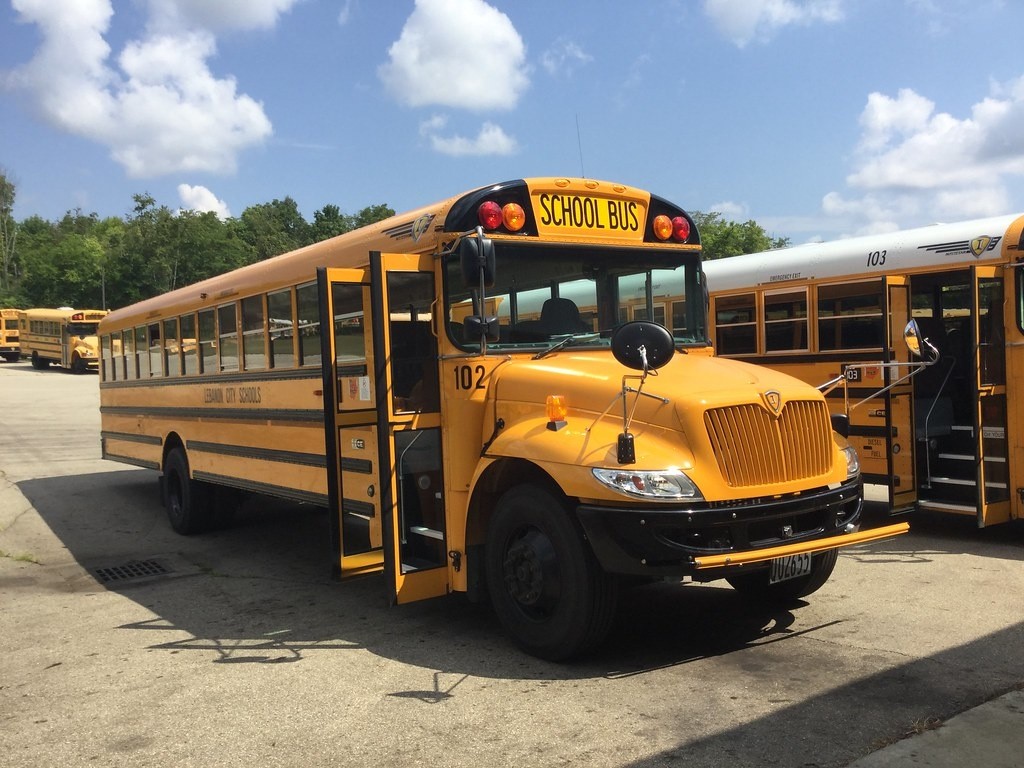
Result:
[541,297,588,337]
[186,320,469,396]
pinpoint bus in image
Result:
[99,176,1024,662]
[0,307,126,374]
